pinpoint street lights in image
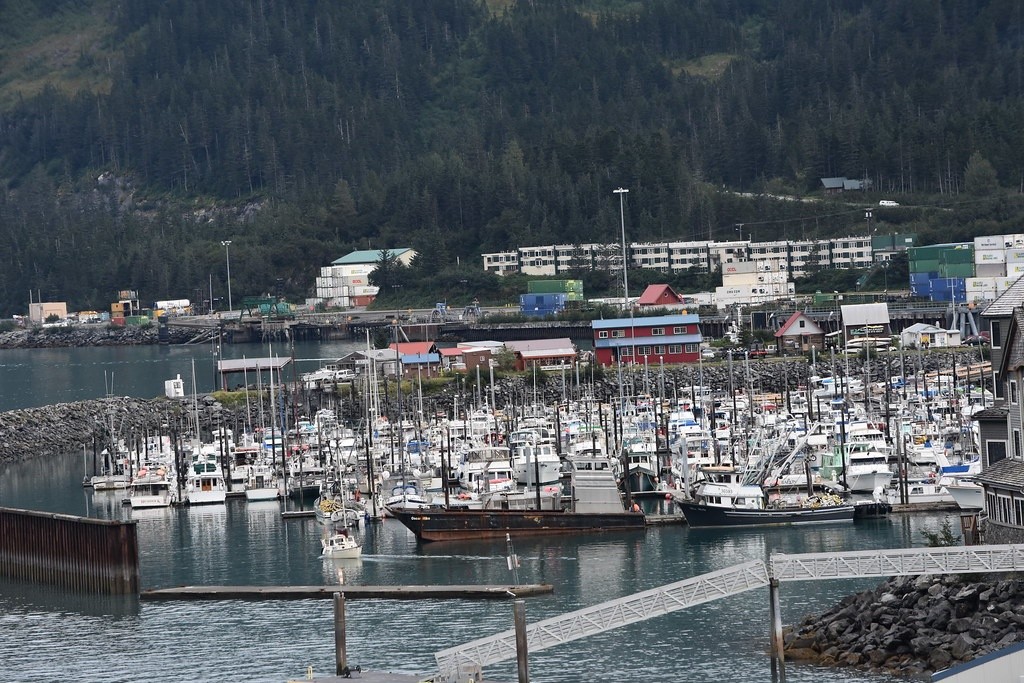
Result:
[833,289,841,354]
[219,239,235,313]
[613,186,631,311]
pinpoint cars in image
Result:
[961,334,989,347]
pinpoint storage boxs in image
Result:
[306,266,380,307]
[715,258,796,307]
[111,302,192,325]
[519,280,584,317]
[907,234,1024,302]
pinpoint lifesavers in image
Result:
[485,433,503,444]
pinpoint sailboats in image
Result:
[76,298,994,560]
